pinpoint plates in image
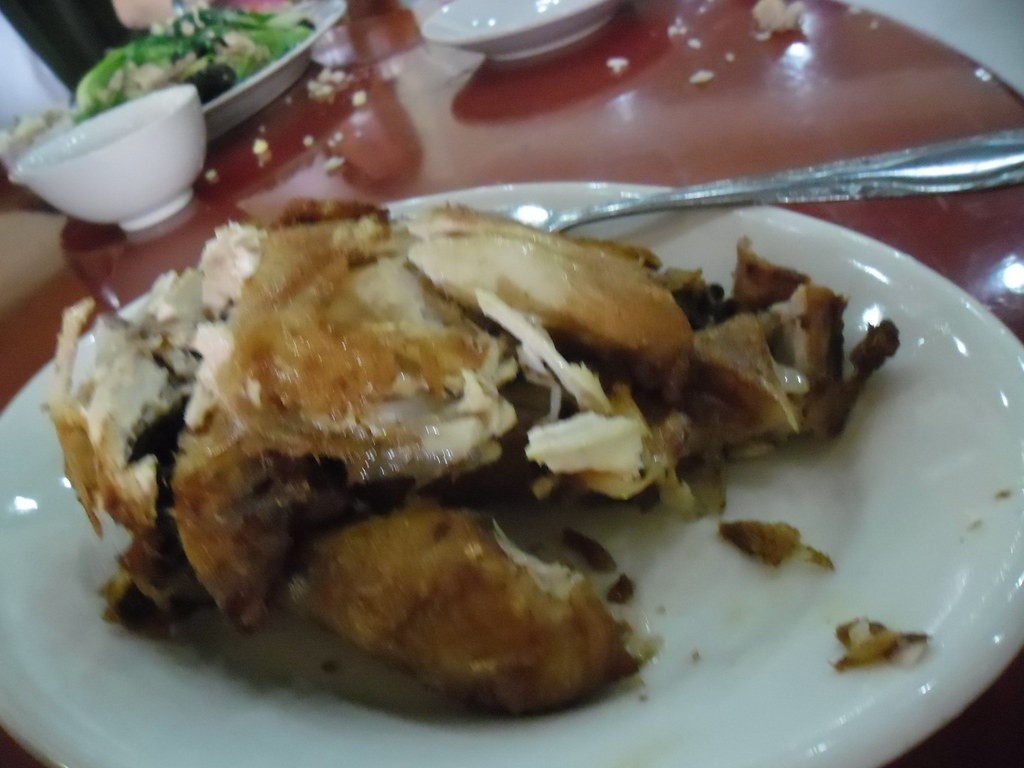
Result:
[2,180,1024,768]
[42,0,349,142]
[421,0,617,61]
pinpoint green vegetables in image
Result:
[69,4,315,122]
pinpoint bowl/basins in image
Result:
[7,84,208,232]
